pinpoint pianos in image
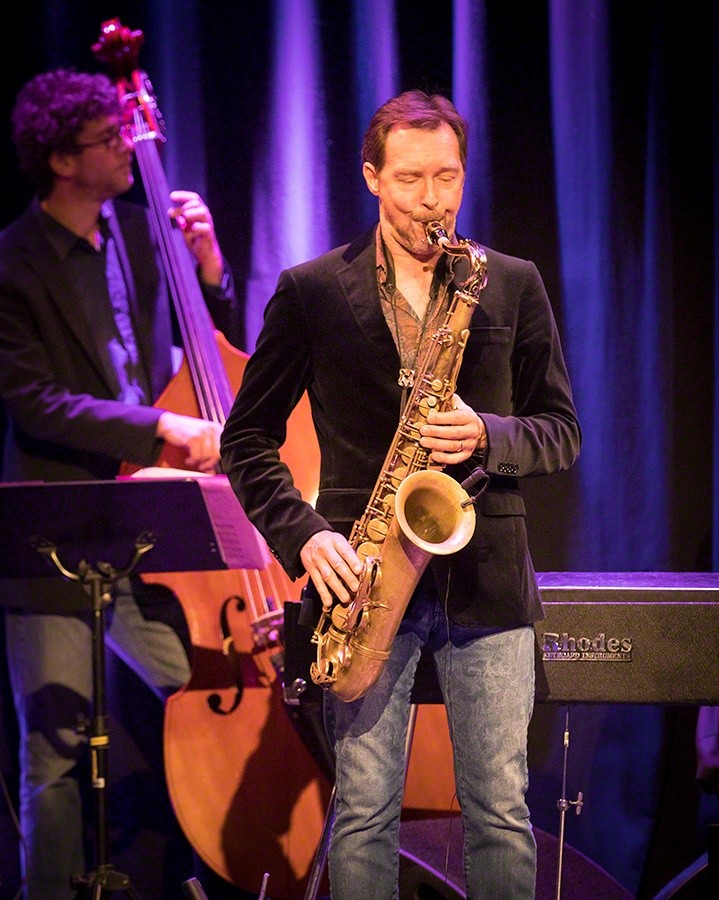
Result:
[528,572,719,708]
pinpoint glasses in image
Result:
[76,123,122,149]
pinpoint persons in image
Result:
[219,87,582,900]
[0,69,239,900]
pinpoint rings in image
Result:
[457,440,462,452]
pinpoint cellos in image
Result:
[89,11,463,900]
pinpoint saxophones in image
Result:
[307,219,494,707]
